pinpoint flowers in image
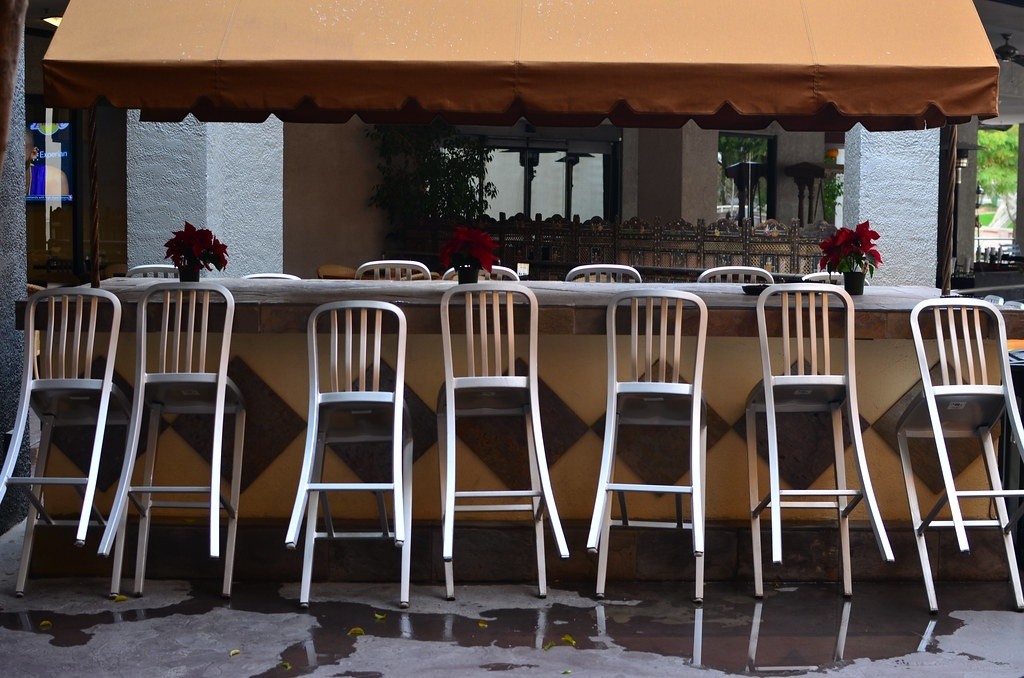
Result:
[818,222,883,280]
[440,226,499,272]
[163,219,230,273]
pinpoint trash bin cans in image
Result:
[951,272,975,297]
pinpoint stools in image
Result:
[441,266,520,281]
[126,264,180,277]
[586,288,711,604]
[436,282,571,601]
[97,281,252,598]
[354,259,432,279]
[745,281,896,596]
[0,287,120,602]
[695,266,775,283]
[801,272,872,285]
[283,300,413,615]
[895,293,1024,619]
[565,263,642,283]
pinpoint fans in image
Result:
[990,33,1024,67]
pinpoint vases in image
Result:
[458,260,478,284]
[179,262,200,282]
[845,272,866,294]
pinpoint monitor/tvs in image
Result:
[24,92,75,206]
